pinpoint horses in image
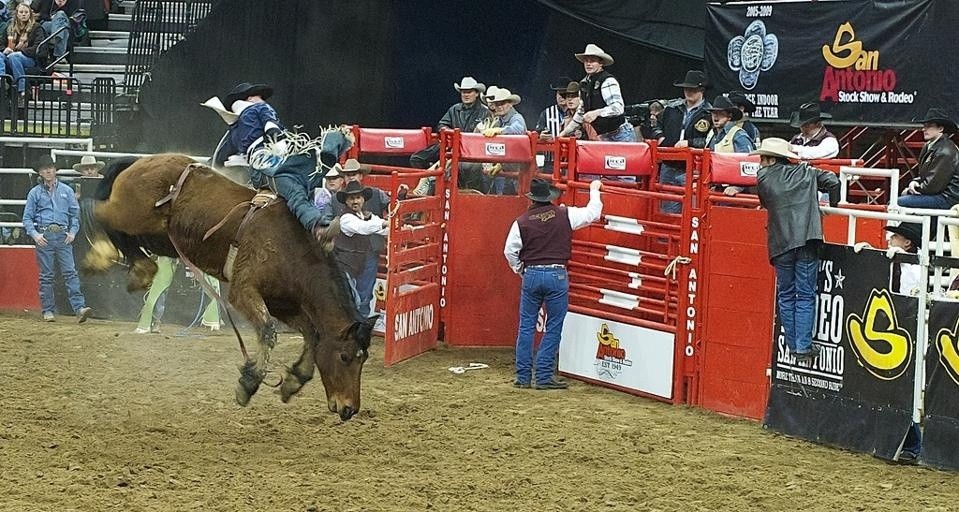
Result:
[81,153,380,421]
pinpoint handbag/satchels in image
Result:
[70,9,90,46]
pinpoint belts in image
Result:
[527,263,567,269]
[40,224,68,232]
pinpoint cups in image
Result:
[8,36,14,49]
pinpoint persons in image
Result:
[503,176,605,389]
[200,81,389,317]
[22,154,93,323]
[0,0,112,120]
[852,219,920,461]
[402,39,959,258]
[747,138,840,361]
[69,154,105,200]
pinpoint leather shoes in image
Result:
[513,380,531,389]
[536,379,567,390]
[797,346,822,361]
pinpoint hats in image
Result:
[337,180,373,203]
[575,44,614,66]
[73,155,105,171]
[673,70,715,91]
[453,76,486,94]
[908,108,959,133]
[703,91,756,121]
[324,159,371,179]
[883,222,922,248]
[224,82,274,112]
[523,178,561,203]
[31,154,62,172]
[747,137,802,160]
[480,86,522,106]
[789,103,833,128]
[549,76,580,99]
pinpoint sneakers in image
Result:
[43,312,55,322]
[76,307,92,323]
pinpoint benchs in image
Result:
[26,1,224,126]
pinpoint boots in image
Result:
[316,216,339,252]
